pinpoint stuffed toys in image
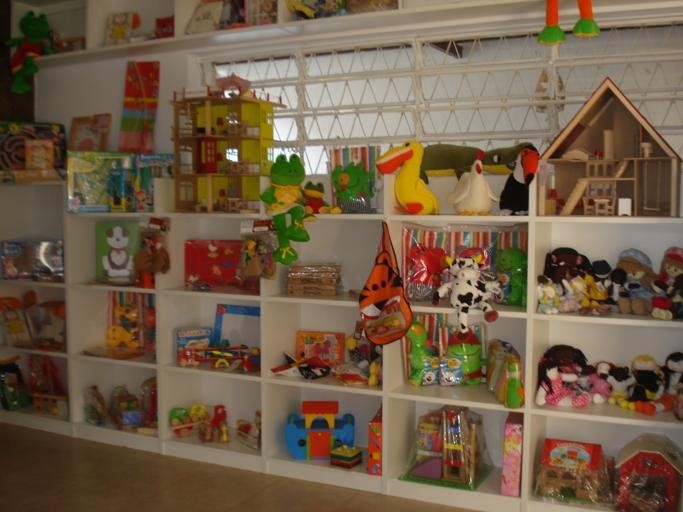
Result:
[433,256,501,338]
[259,154,310,265]
[535,344,683,423]
[536,247,683,321]
[134,229,171,275]
[3,12,53,94]
[538,0,599,45]
[375,139,539,216]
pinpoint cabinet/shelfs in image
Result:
[0,0,683,512]
